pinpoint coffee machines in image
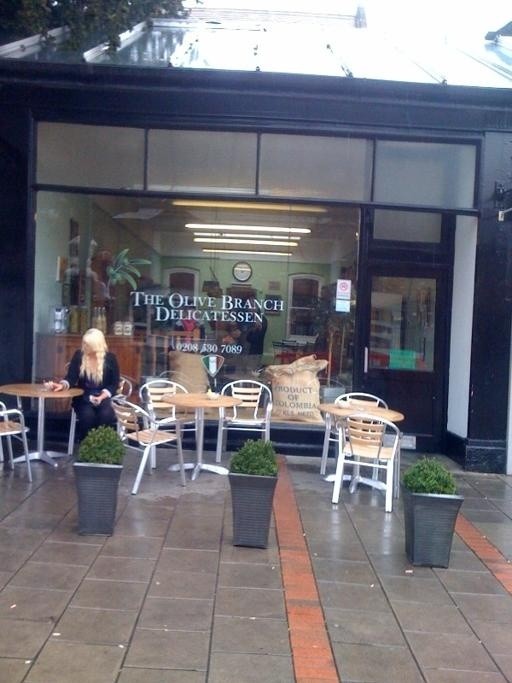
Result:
[48,302,69,333]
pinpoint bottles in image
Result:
[93,305,107,333]
[113,320,123,336]
[124,321,133,336]
[80,304,88,335]
[70,304,79,334]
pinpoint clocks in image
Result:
[231,260,253,282]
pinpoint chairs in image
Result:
[214,378,274,466]
[333,412,402,514]
[1,402,34,482]
[320,393,389,477]
[139,381,202,471]
[111,397,188,493]
[67,376,135,456]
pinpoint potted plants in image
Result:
[398,452,468,572]
[225,434,285,551]
[69,419,131,539]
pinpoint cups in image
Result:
[43,377,54,391]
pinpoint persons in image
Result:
[239,296,267,355]
[175,303,205,353]
[51,330,119,445]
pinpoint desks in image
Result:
[318,403,405,492]
[2,383,84,468]
[162,392,244,481]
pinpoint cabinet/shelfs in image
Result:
[35,327,147,407]
